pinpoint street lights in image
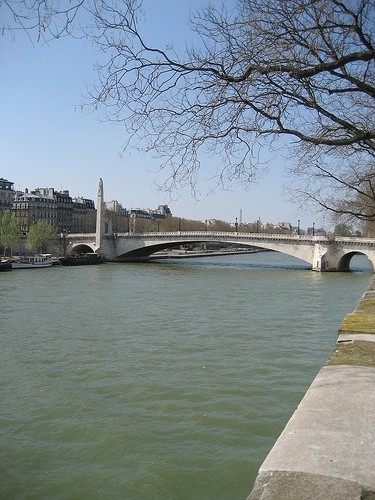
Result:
[235,217,238,231]
[256,220,259,232]
[205,219,207,231]
[157,222,161,231]
[178,217,181,231]
[312,223,315,236]
[297,219,300,235]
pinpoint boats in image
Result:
[9,254,53,269]
[58,253,102,265]
